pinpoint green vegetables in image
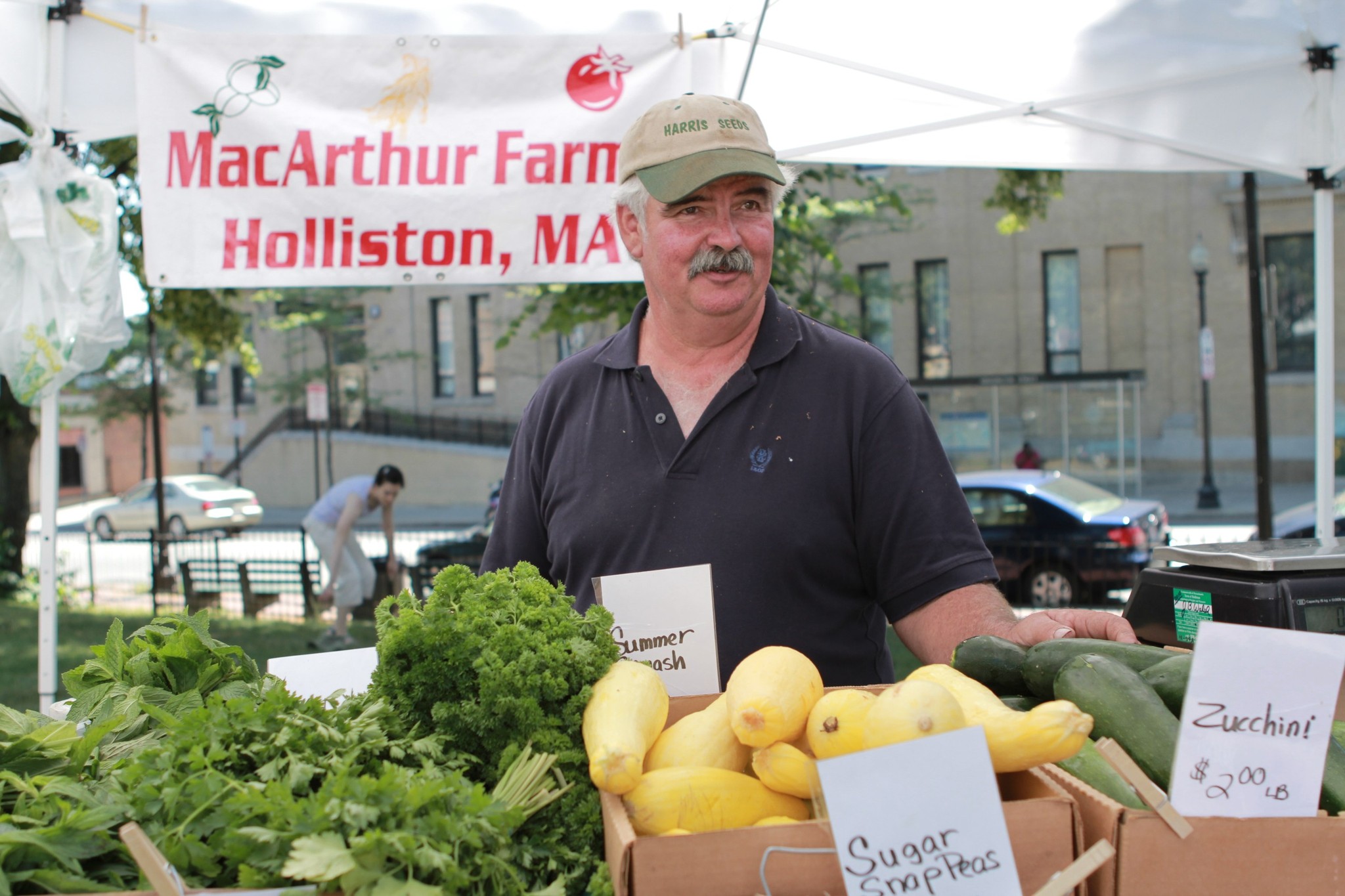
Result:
[0,560,626,896]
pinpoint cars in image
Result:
[954,467,1173,612]
[84,472,264,543]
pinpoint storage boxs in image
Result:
[600,681,1345,896]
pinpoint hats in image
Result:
[617,92,786,206]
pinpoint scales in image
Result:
[1122,536,1345,651]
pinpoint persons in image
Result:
[1015,442,1042,469]
[301,464,405,647]
[477,93,1144,694]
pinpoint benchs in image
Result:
[178,557,321,617]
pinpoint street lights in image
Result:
[1187,243,1223,511]
